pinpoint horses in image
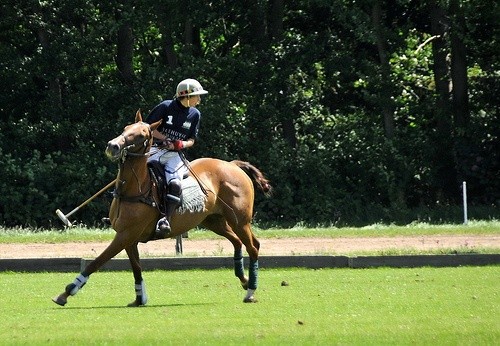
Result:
[51,108,272,307]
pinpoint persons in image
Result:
[101,78,209,236]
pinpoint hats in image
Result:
[176,78,208,97]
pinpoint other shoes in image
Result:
[160,223,170,232]
[102,218,111,225]
